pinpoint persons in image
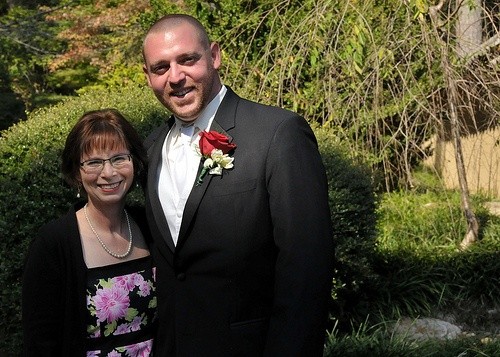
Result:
[22,112,160,357]
[134,13,338,357]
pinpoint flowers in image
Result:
[198,130,238,179]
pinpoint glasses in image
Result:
[80,153,133,171]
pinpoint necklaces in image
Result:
[83,202,134,258]
